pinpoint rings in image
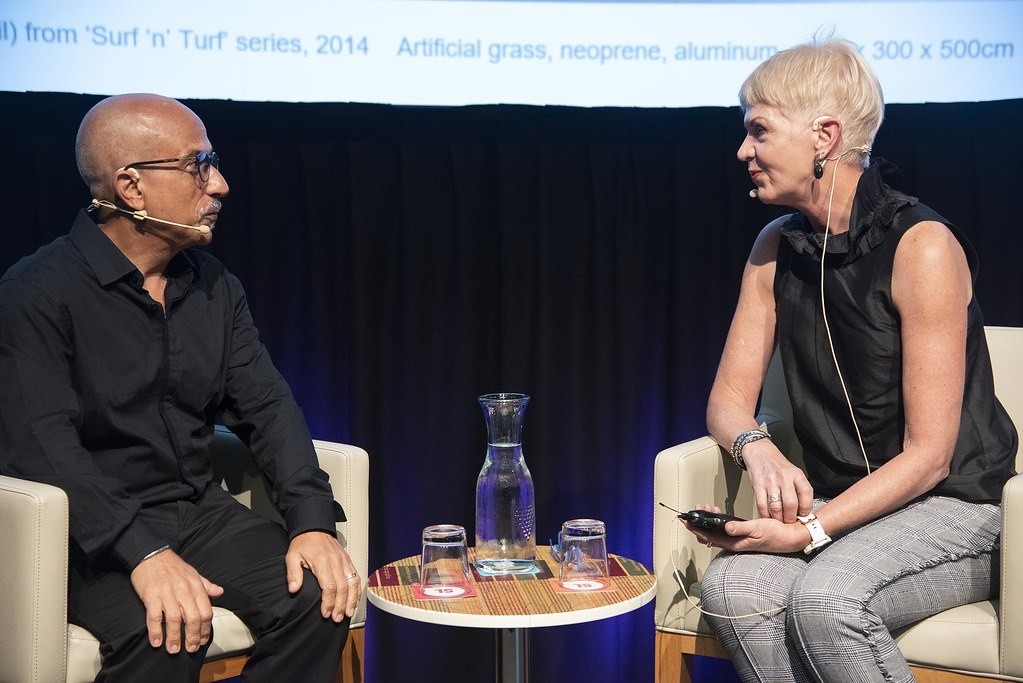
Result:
[344,573,356,580]
[767,494,782,503]
[706,542,712,548]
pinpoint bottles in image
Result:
[475,392,536,574]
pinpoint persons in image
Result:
[677,39,1019,683]
[0,94,361,683]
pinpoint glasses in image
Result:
[124,151,220,182]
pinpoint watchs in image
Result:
[796,513,832,556]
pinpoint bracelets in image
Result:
[140,545,171,562]
[731,429,771,472]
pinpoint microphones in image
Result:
[749,189,758,198]
[101,203,211,234]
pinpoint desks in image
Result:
[367,544,657,683]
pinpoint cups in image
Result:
[420,524,473,598]
[558,518,611,591]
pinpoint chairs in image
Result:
[0,438,369,683]
[654,324,1023,683]
